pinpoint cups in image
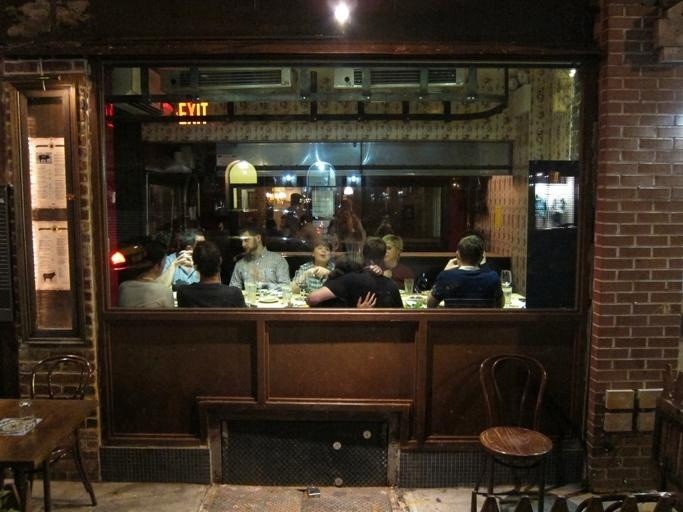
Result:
[500,287,512,305]
[280,282,293,303]
[535,178,574,228]
[403,277,413,296]
[499,269,511,287]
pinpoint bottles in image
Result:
[318,221,324,230]
[16,396,37,434]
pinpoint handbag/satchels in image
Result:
[414,267,440,292]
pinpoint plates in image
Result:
[503,298,526,308]
[256,290,306,308]
[399,300,426,309]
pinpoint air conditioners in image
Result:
[161,67,298,96]
[333,66,468,95]
[107,46,162,117]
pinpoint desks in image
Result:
[0,397,98,511]
[270,251,510,265]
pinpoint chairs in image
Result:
[286,255,312,282]
[172,287,526,309]
[474,353,557,512]
[28,353,97,511]
[231,252,243,262]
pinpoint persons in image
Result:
[426,234,505,309]
[118,192,414,310]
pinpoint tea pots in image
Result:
[243,262,257,304]
